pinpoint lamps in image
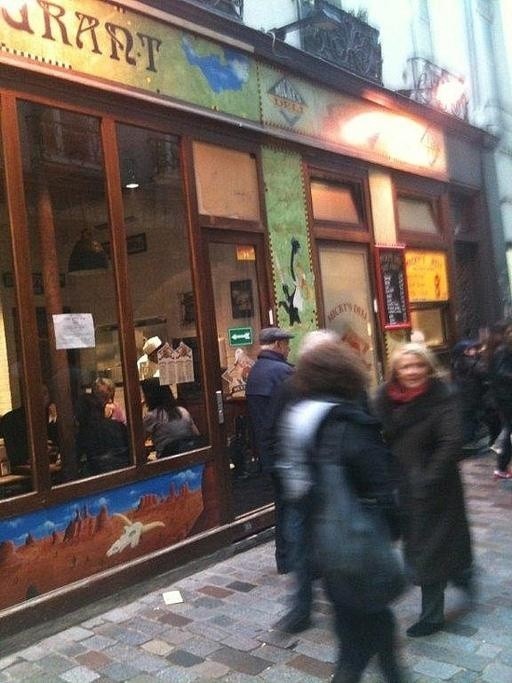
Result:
[266,9,344,42]
[67,207,112,276]
[395,76,464,105]
[236,244,256,261]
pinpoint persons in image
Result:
[142,335,168,378]
[0,373,205,494]
[242,313,512,682]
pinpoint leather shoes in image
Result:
[275,607,311,634]
[407,620,445,637]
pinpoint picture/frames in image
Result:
[101,232,147,259]
[230,280,254,319]
[4,271,66,288]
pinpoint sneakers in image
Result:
[494,468,512,480]
[488,443,503,455]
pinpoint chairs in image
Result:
[156,436,206,458]
[0,438,27,498]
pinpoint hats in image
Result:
[451,340,483,356]
[142,335,163,356]
[258,327,295,345]
